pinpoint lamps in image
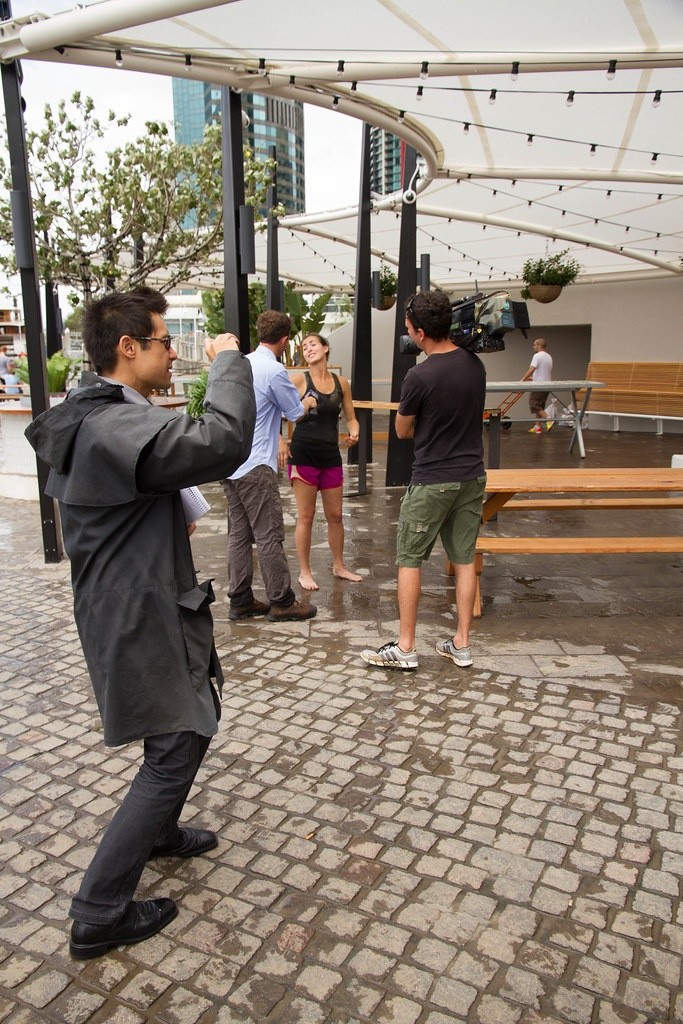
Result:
[238,204,256,276]
[417,254,430,292]
[371,269,381,309]
[279,280,286,314]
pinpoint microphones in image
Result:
[450,298,468,308]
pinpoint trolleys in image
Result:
[483,378,532,430]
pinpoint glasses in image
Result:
[132,335,172,350]
[406,299,426,332]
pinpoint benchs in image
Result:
[474,498,683,552]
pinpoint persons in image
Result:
[278,333,362,590]
[520,338,556,434]
[218,311,317,622]
[25,285,256,959]
[361,292,487,669]
[0,346,23,402]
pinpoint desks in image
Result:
[443,468,682,619]
[485,381,608,458]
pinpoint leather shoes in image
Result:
[70,898,179,960]
[151,823,219,857]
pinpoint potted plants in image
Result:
[520,253,579,303]
[349,256,398,311]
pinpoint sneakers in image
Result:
[268,599,317,622]
[360,641,419,668]
[528,426,542,433]
[436,636,473,667]
[229,598,268,621]
[545,420,555,432]
